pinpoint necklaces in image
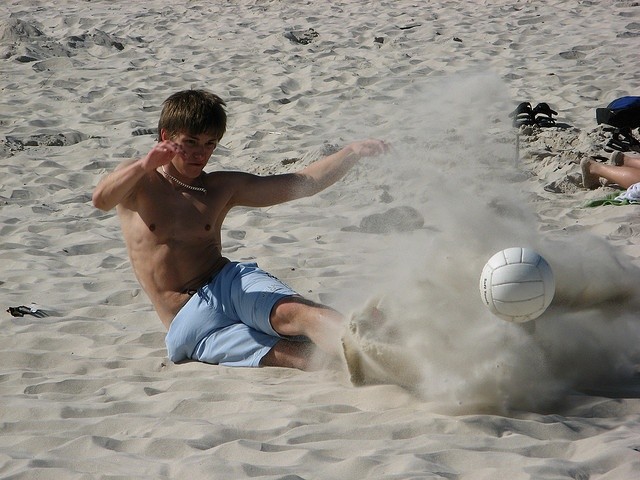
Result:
[162,165,207,196]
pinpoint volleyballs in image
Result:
[481,246,556,323]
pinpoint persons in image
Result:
[93,89,392,373]
[580,150,640,192]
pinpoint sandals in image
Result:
[533,102,557,126]
[508,102,534,129]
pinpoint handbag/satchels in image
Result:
[596,96,640,129]
